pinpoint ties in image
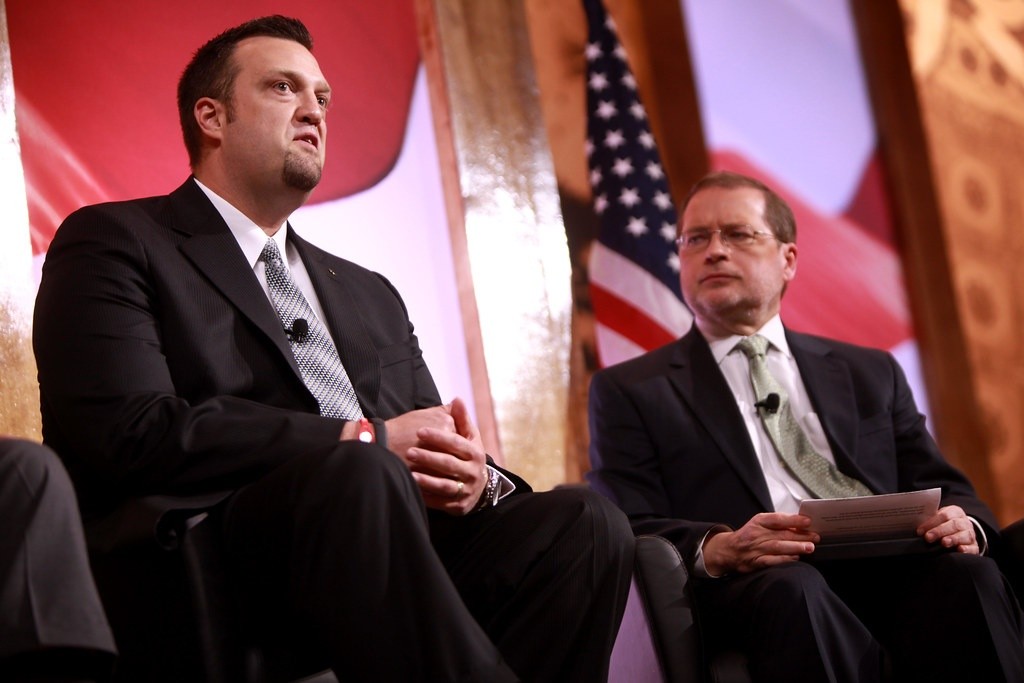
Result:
[254,244,364,420]
[736,337,874,500]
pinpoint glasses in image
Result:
[674,224,771,253]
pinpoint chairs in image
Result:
[182,510,339,683]
[634,519,1024,683]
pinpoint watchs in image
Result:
[478,468,502,510]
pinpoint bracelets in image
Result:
[356,417,387,448]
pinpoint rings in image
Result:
[456,480,464,495]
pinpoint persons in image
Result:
[586,167,1024,683]
[32,13,633,683]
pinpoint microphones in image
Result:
[292,319,308,343]
[766,393,780,413]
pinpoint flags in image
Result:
[584,0,694,367]
